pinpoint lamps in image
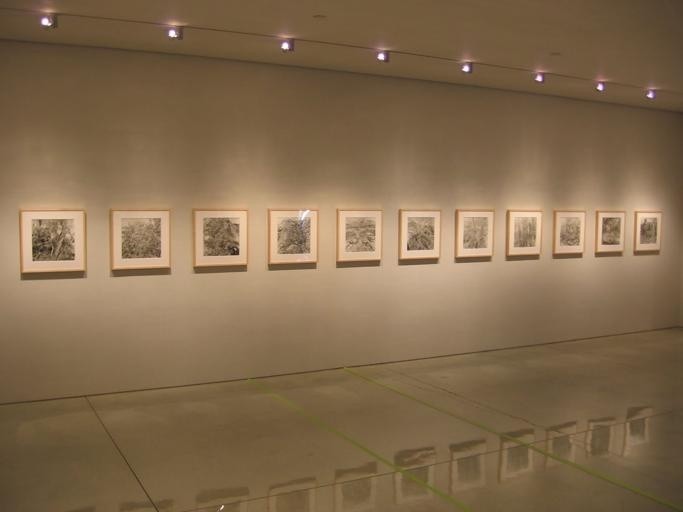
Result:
[279,40,294,54]
[534,71,545,86]
[167,28,184,39]
[461,61,474,75]
[39,12,62,29]
[377,50,391,62]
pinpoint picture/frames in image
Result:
[108,210,173,273]
[18,209,89,278]
[452,211,496,261]
[392,447,440,504]
[504,210,542,260]
[550,209,586,258]
[191,210,250,271]
[336,210,384,267]
[268,209,319,267]
[447,437,488,492]
[329,461,380,507]
[497,428,536,483]
[624,409,649,451]
[544,421,578,471]
[397,210,442,262]
[591,211,626,255]
[585,414,616,463]
[632,210,663,254]
[267,477,320,506]
[195,486,251,506]
